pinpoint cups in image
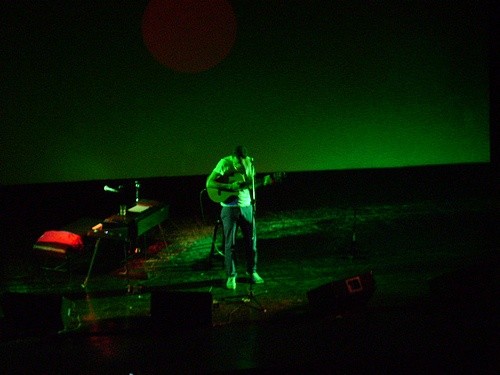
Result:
[119,204,126,215]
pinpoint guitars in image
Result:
[206,172,286,202]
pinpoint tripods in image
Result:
[221,166,267,313]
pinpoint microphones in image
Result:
[104,185,119,192]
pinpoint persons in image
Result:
[206,145,271,290]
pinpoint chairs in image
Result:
[200,189,239,270]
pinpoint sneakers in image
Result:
[251,273,264,284]
[227,277,236,290]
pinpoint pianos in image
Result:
[87,199,169,243]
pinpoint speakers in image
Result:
[151,290,212,336]
[306,273,376,312]
[0,292,64,342]
[423,260,500,316]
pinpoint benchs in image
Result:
[32,215,109,283]
